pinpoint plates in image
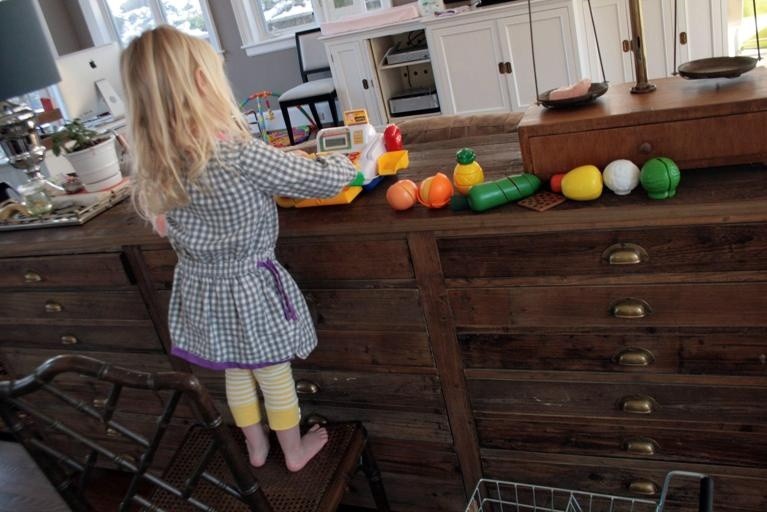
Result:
[537,83,609,107]
[677,56,758,79]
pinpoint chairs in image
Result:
[278,28,338,146]
[0,353,391,512]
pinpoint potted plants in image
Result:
[50,118,123,192]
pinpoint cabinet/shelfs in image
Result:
[313,32,404,126]
[673,0,727,74]
[573,1,678,86]
[420,0,583,115]
[0,66,767,512]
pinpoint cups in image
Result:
[17,179,54,220]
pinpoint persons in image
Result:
[119,24,357,473]
